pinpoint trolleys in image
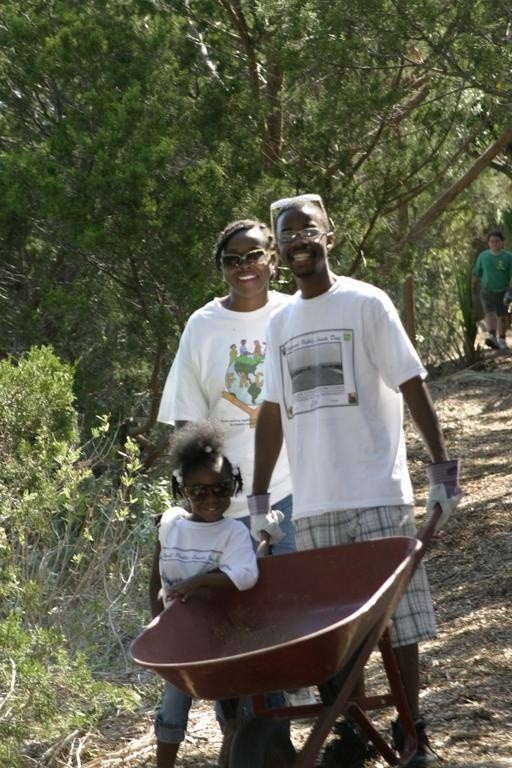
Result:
[122,501,426,768]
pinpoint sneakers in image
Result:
[485,335,499,349]
[390,716,439,763]
[498,339,509,349]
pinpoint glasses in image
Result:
[276,226,324,242]
[177,479,235,500]
[269,194,329,235]
[219,248,275,268]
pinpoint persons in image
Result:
[155,217,303,740]
[146,418,263,767]
[502,277,512,312]
[245,189,465,768]
[470,232,512,352]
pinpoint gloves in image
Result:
[246,492,287,546]
[426,459,463,529]
